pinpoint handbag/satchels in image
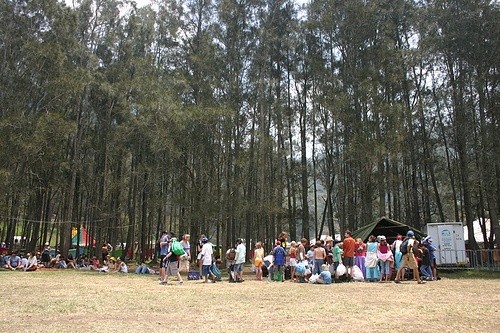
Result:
[399,241,407,254]
[335,263,347,276]
[295,261,306,274]
[309,273,319,284]
[172,240,185,256]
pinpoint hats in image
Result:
[334,239,339,243]
[407,231,414,236]
[230,248,235,253]
[111,257,115,260]
[380,236,386,240]
[276,241,281,244]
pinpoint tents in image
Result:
[352,216,427,242]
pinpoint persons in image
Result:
[40,242,52,267]
[13,239,20,248]
[0,250,20,271]
[19,252,37,271]
[391,230,441,284]
[102,243,111,261]
[197,234,218,283]
[225,238,246,282]
[307,236,342,284]
[342,230,355,282]
[158,231,183,284]
[0,242,8,256]
[178,234,190,273]
[356,235,393,283]
[135,266,159,275]
[278,232,286,251]
[252,238,308,282]
[49,250,102,270]
[101,255,127,273]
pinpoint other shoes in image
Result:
[160,281,168,284]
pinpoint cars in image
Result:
[51,248,82,260]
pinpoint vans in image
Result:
[13,236,30,246]
